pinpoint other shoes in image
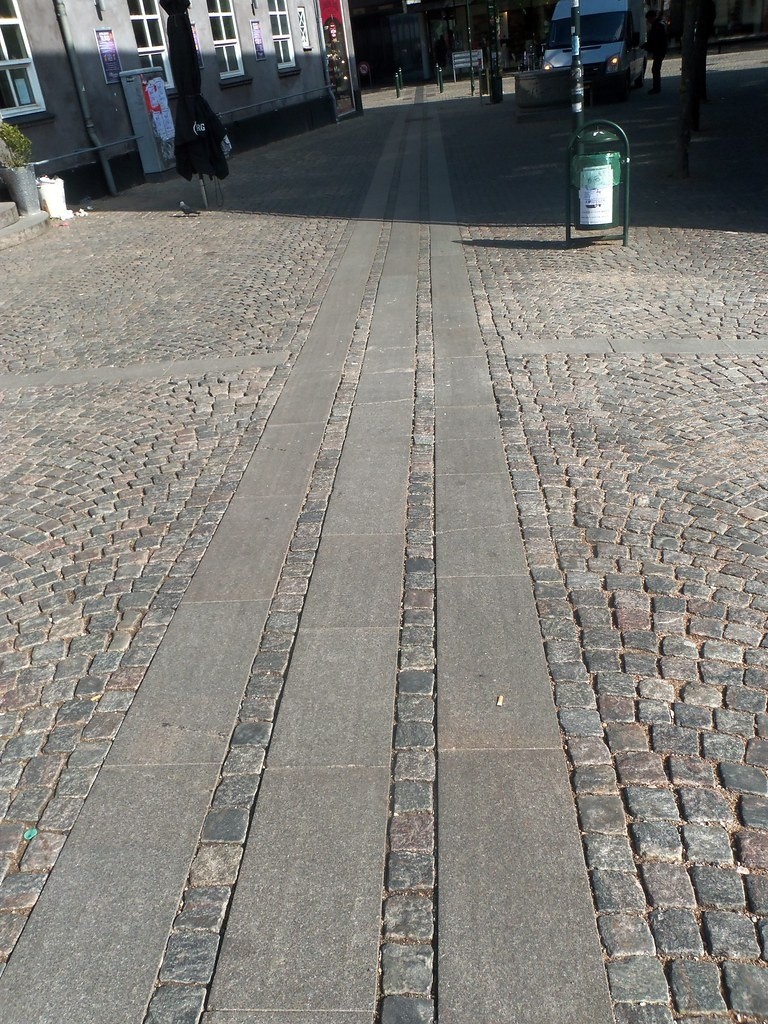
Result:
[648,87,661,95]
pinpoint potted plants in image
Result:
[0,120,41,216]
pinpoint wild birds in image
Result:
[179,200,201,217]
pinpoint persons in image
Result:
[641,10,665,95]
[670,0,717,103]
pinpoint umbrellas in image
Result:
[159,0,229,209]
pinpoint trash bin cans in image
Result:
[564,119,632,247]
[489,75,503,105]
[478,67,492,101]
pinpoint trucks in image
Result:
[541,0,648,102]
